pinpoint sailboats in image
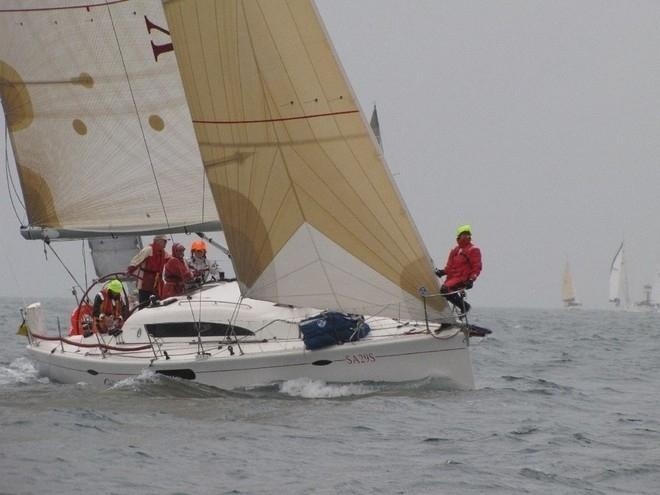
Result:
[561,240,659,314]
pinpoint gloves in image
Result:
[466,281,473,289]
[435,268,442,278]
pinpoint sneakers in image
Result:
[460,304,470,318]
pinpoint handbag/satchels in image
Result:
[299,307,370,350]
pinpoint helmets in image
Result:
[192,240,208,250]
[107,279,122,293]
[172,242,185,251]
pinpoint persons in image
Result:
[93,279,130,331]
[125,235,171,310]
[158,242,194,301]
[184,239,220,288]
[434,223,483,331]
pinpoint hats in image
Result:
[153,234,171,240]
[457,224,471,237]
